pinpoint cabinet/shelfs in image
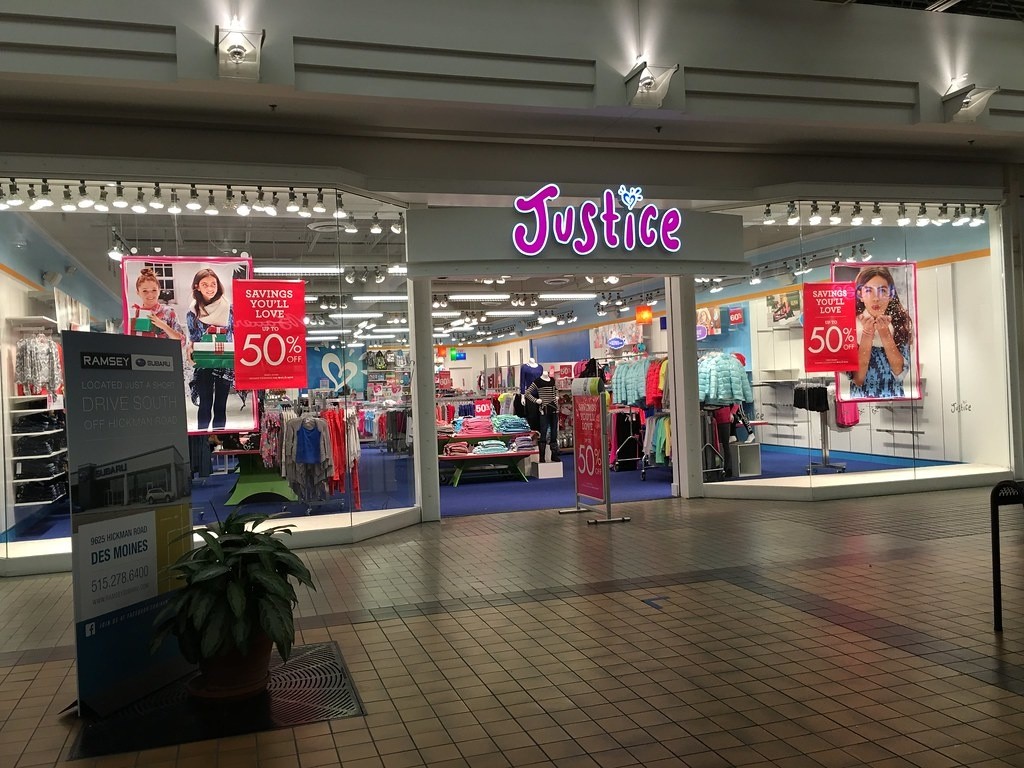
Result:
[608,404,639,414]
[366,351,411,403]
[4,315,68,508]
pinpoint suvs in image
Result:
[146,489,174,503]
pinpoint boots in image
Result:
[539,440,546,462]
[550,441,562,462]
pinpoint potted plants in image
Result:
[156,495,317,702]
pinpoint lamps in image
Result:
[762,199,987,227]
[0,176,405,234]
[632,54,655,92]
[949,80,971,108]
[213,14,256,66]
[300,243,876,350]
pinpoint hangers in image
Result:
[616,346,730,361]
[794,380,825,388]
[653,408,671,418]
[301,405,317,422]
[432,397,475,406]
[263,401,294,412]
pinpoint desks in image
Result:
[211,444,301,506]
[435,430,539,488]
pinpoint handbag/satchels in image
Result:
[542,401,557,415]
[366,349,407,371]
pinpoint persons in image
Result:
[524,370,561,463]
[841,267,914,399]
[131,268,186,347]
[186,269,248,431]
[520,358,543,430]
